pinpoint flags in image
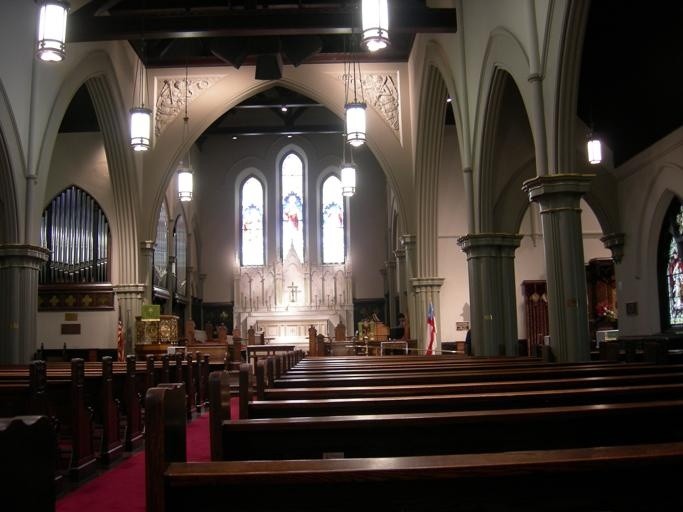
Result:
[116,298,125,362]
[424,298,438,355]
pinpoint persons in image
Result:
[398,312,409,340]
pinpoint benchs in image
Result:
[0,348,683,512]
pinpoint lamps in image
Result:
[129,34,152,152]
[340,124,357,198]
[344,1,370,148]
[35,0,66,64]
[584,127,602,165]
[359,0,392,54]
[176,64,193,203]
